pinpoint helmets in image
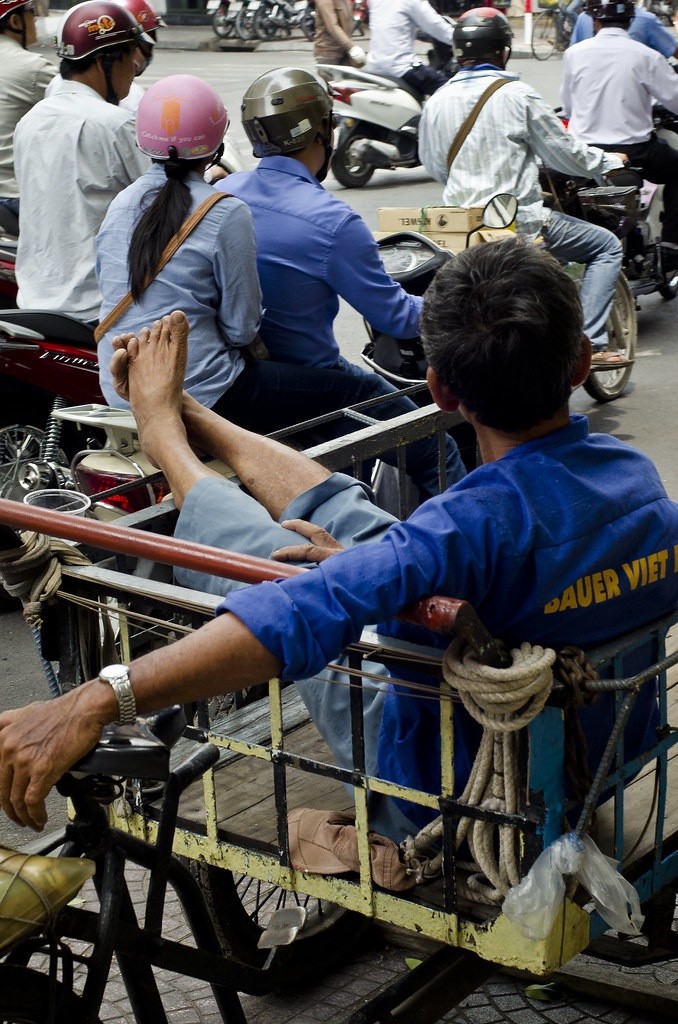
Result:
[56,0,157,61]
[0,0,31,20]
[581,0,636,22]
[241,68,339,157]
[453,7,514,57]
[106,0,168,33]
[133,76,229,161]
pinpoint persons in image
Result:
[211,66,467,505]
[570,0,678,60]
[0,0,60,212]
[314,0,366,79]
[560,0,678,271]
[0,239,678,846]
[14,0,229,324]
[363,0,455,95]
[418,7,635,365]
[110,0,167,111]
[95,73,361,437]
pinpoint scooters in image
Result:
[206,0,317,42]
[607,64,677,302]
[313,14,463,190]
[0,147,520,622]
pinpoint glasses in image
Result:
[20,7,37,16]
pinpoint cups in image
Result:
[23,489,91,547]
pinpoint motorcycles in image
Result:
[533,104,639,405]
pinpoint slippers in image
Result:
[591,351,626,364]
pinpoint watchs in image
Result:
[99,664,136,726]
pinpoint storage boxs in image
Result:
[377,206,516,256]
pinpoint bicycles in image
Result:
[530,0,584,61]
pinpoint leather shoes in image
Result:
[661,246,678,279]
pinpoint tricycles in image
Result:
[0,381,678,1024]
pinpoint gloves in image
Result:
[347,45,366,68]
